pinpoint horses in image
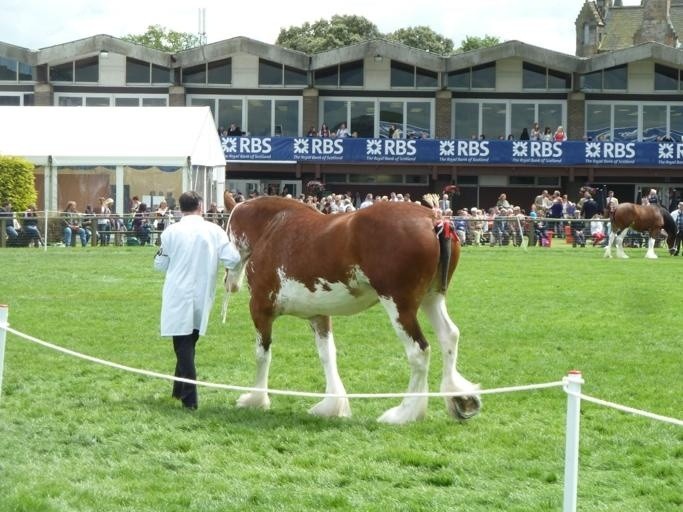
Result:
[604,203,679,259]
[223,190,482,424]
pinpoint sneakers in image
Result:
[182,393,198,407]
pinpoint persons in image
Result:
[152,188,244,412]
